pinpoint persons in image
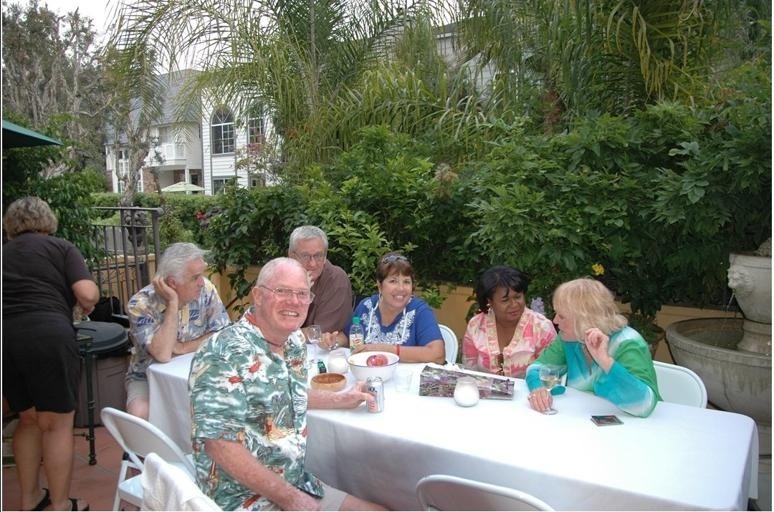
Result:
[524,279,660,417]
[288,224,350,343]
[317,251,445,365]
[123,242,232,474]
[460,266,557,380]
[2,196,100,511]
[185,257,391,511]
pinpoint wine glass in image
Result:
[306,325,322,363]
[537,363,560,417]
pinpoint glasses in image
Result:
[293,251,325,263]
[258,284,316,305]
[381,255,408,264]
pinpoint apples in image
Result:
[366,354,387,367]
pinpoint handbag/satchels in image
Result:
[419,366,514,400]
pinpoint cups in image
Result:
[453,376,480,405]
[72,303,82,324]
[328,351,348,375]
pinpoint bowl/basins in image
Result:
[346,351,400,384]
[310,372,344,391]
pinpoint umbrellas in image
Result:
[2,120,61,151]
[155,181,204,193]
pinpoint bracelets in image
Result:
[396,343,399,356]
[333,336,339,349]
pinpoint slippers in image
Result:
[70,498,89,511]
[33,488,50,511]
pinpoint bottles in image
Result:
[348,317,364,350]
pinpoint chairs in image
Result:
[416,474,552,510]
[438,323,459,364]
[99,407,198,511]
[139,453,221,511]
[653,360,708,408]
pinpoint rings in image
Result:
[527,397,531,400]
[530,394,535,398]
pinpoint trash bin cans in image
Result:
[74,322,130,428]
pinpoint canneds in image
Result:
[366,376,384,414]
